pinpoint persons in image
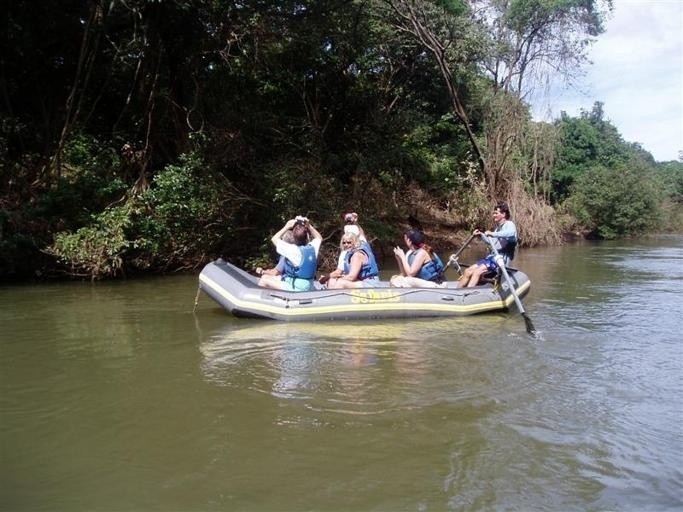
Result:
[452,203,519,288]
[255,212,449,292]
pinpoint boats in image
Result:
[198,257,532,322]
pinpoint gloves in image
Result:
[319,275,330,284]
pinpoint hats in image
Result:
[406,229,422,244]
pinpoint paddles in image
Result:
[487,236,536,332]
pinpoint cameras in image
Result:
[341,213,353,223]
[295,217,307,226]
